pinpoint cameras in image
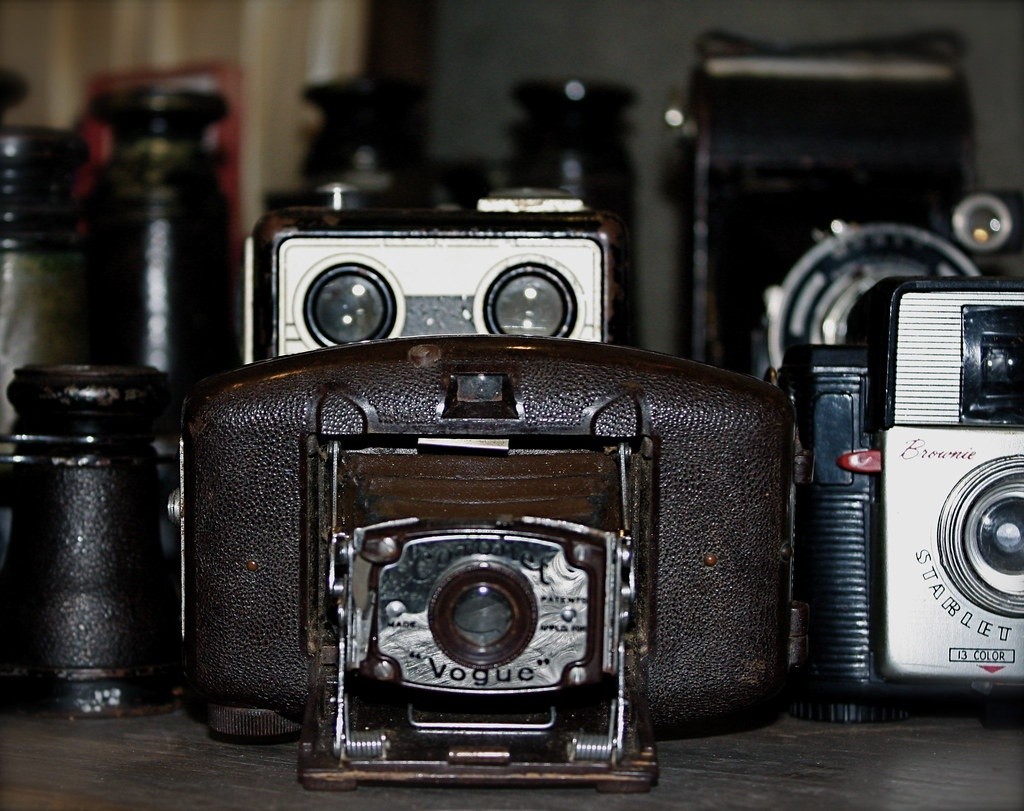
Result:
[0,30,1024,796]
